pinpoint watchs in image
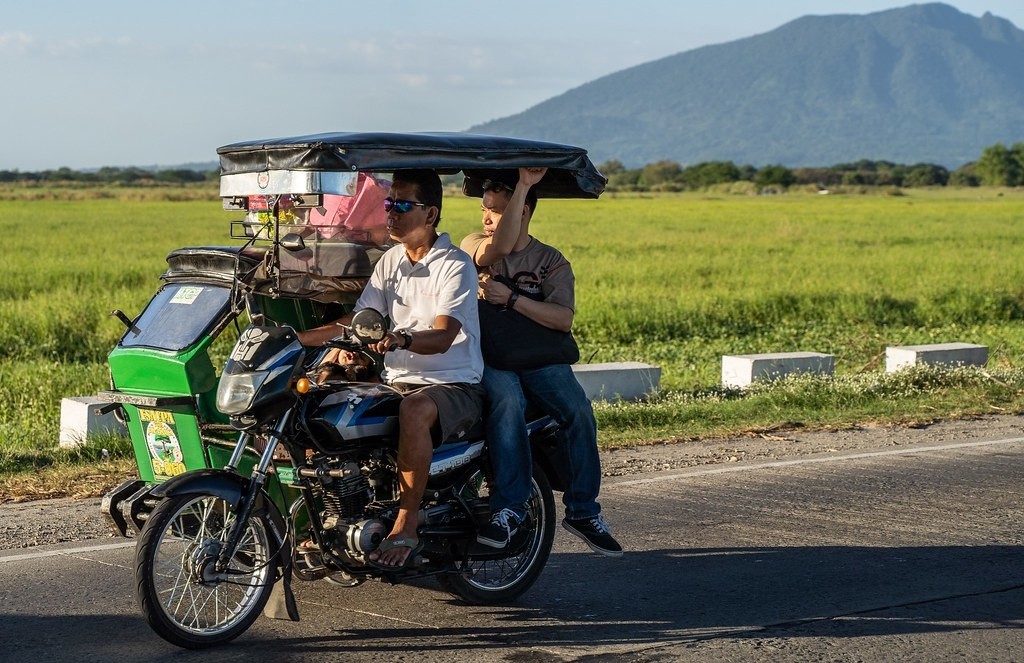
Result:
[398,329,412,350]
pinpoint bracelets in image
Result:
[506,292,519,310]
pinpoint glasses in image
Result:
[384,197,431,214]
[481,178,516,193]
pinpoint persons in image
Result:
[460,167,625,557]
[296,168,484,567]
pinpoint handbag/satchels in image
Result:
[478,273,579,370]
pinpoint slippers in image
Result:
[295,539,319,554]
[368,537,425,571]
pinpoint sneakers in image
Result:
[477,506,520,550]
[562,513,625,558]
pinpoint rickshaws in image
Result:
[94,133,608,650]
